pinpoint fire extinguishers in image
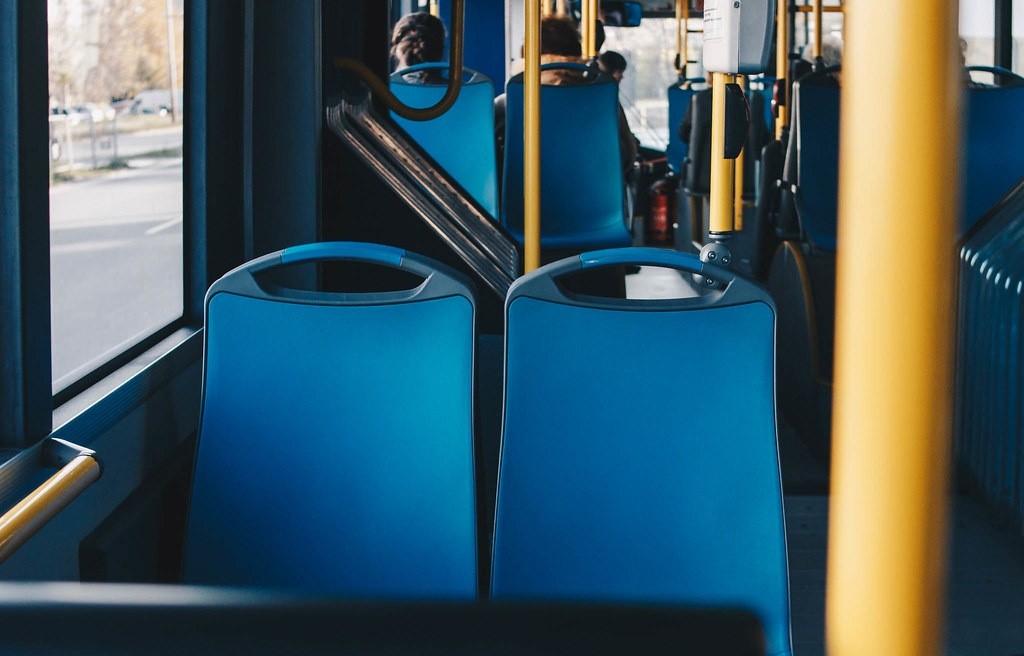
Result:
[643,168,676,242]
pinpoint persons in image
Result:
[389,10,452,85]
[802,36,842,70]
[494,16,640,231]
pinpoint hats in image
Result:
[522,17,581,57]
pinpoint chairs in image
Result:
[960,65,1024,238]
[664,77,707,172]
[499,61,636,259]
[486,246,795,656]
[795,64,840,252]
[749,77,776,161]
[388,61,499,221]
[175,240,481,600]
